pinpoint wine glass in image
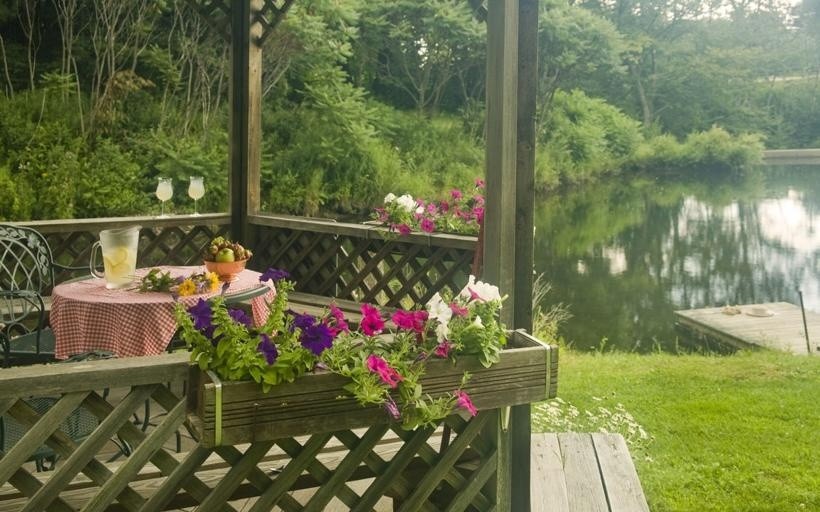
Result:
[188,175,205,217]
[155,178,175,219]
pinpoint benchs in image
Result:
[1,424,482,511]
[286,292,400,332]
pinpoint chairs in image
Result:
[0,222,112,471]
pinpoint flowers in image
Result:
[369,179,484,236]
[171,267,507,429]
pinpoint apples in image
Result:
[215,248,235,262]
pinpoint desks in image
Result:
[51,264,273,463]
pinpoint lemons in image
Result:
[102,249,130,273]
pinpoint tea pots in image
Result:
[90,225,144,289]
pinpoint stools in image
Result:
[529,431,649,512]
